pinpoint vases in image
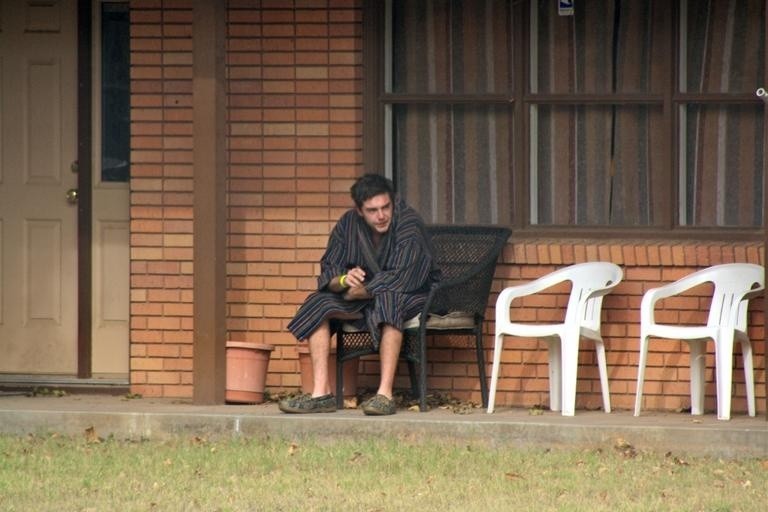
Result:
[223,341,356,403]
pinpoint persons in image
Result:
[277,171,442,417]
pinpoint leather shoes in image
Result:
[362,394,397,415]
[278,392,337,414]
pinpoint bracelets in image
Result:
[339,275,347,288]
